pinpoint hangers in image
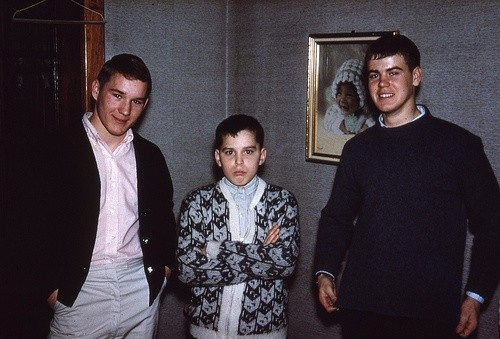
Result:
[11,0,106,24]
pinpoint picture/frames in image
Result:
[305,30,400,165]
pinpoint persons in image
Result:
[322,59,377,135]
[312,34,500,339]
[175,113,299,339]
[10,53,170,338]
